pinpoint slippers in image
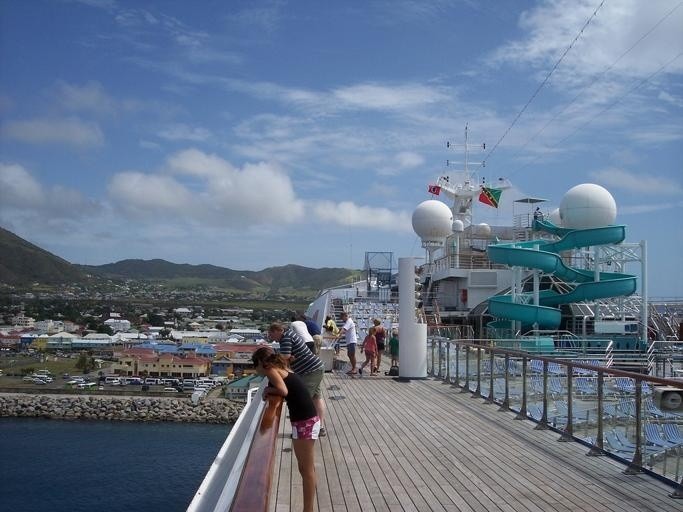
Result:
[346,371,358,374]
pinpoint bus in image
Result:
[106,376,181,393]
[183,378,217,392]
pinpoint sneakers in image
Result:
[318,426,326,438]
[358,367,381,376]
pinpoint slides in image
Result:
[486,220,640,351]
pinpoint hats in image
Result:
[338,312,346,319]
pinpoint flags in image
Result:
[428,184,441,195]
[479,187,503,208]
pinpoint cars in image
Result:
[23,370,53,385]
[64,373,105,390]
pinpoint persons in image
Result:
[534,206,542,220]
[269,321,326,436]
[324,316,340,354]
[252,346,321,512]
[359,327,379,376]
[302,316,322,354]
[290,317,315,351]
[372,319,385,372]
[338,312,357,374]
[389,330,399,367]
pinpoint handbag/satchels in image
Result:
[384,366,398,376]
